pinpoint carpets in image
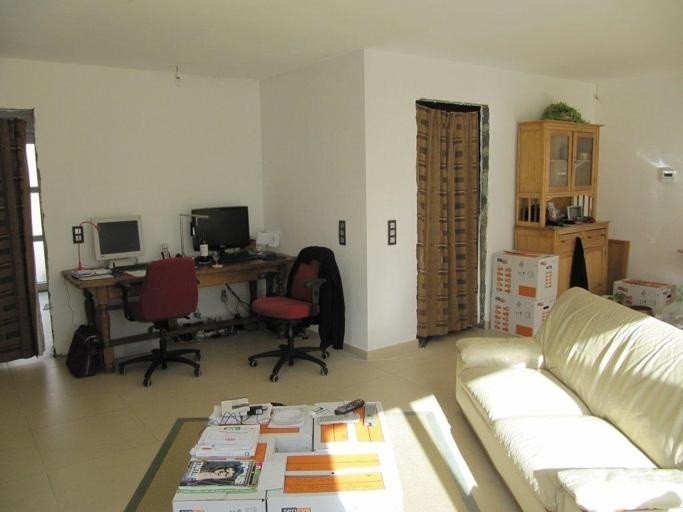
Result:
[124,410,481,512]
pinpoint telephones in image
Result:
[161,244,170,259]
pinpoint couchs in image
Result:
[455,287,683,512]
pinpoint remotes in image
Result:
[334,399,365,415]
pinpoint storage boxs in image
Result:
[613,278,676,312]
[490,294,556,338]
[493,250,559,300]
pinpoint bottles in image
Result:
[199,230,209,266]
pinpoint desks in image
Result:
[172,402,403,512]
[61,251,295,374]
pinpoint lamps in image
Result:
[179,214,209,269]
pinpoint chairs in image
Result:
[119,257,200,387]
[248,245,345,382]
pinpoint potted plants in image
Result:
[542,102,589,124]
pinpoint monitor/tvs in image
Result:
[191,205,251,252]
[92,214,145,268]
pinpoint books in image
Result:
[173,424,277,494]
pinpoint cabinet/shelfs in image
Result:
[514,120,611,300]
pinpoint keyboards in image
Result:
[124,269,147,277]
[216,253,263,264]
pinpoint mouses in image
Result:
[265,255,279,261]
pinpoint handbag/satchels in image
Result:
[65,324,102,378]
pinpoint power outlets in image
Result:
[222,289,226,301]
